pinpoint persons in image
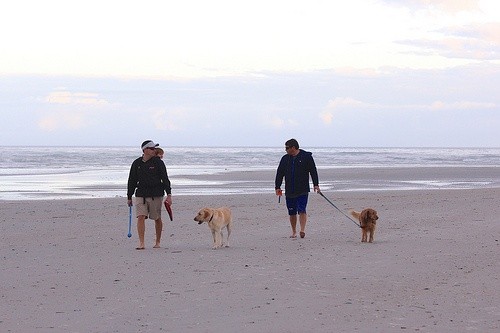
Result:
[127,140,172,249]
[275,139,320,239]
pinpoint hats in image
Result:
[142,141,159,151]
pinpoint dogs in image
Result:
[350,208,379,243]
[194,206,232,250]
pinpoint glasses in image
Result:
[286,146,292,151]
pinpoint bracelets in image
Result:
[168,194,171,196]
